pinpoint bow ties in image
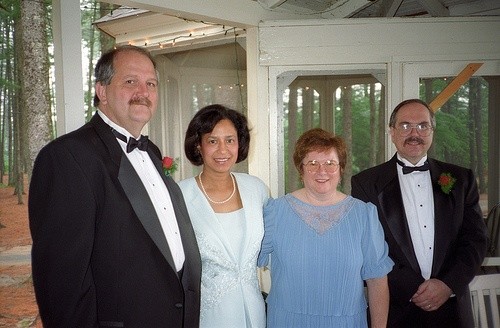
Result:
[395,158,432,174]
[109,127,148,153]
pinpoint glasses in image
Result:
[392,123,433,137]
[301,160,340,174]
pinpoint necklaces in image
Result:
[199,170,235,204]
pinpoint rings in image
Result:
[429,304,432,308]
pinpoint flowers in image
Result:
[161,155,180,179]
[437,172,456,191]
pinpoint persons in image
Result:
[177,104,272,328]
[257,128,394,328]
[351,99,489,328]
[28,43,202,328]
